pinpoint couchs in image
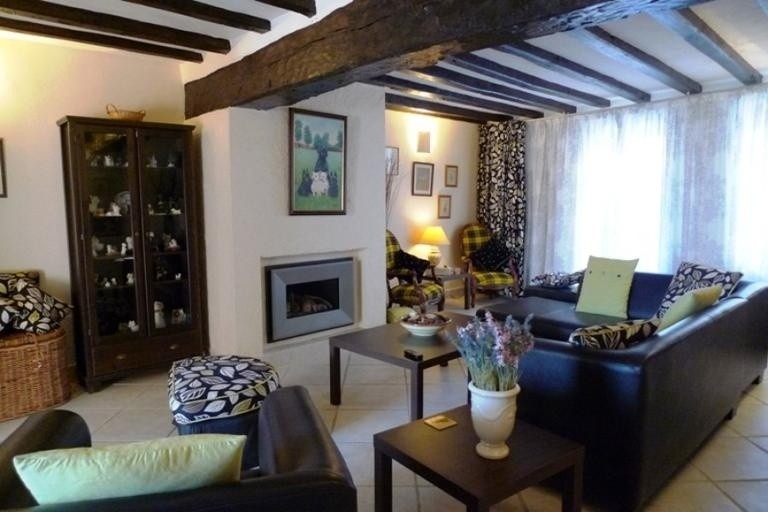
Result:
[524,283,580,302]
[169,354,280,470]
[1,386,358,511]
[467,270,767,512]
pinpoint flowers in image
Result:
[443,310,534,391]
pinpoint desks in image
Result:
[422,272,471,311]
[374,403,586,512]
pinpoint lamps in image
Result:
[417,130,430,154]
[420,226,451,266]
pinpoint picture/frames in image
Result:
[0,137,9,196]
[445,165,458,187]
[439,197,452,219]
[385,146,400,176]
[412,162,435,197]
[287,107,348,216]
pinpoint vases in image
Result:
[466,381,521,460]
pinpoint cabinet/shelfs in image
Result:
[57,115,209,394]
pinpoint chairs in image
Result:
[385,228,446,313]
[458,222,519,307]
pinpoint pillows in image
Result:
[1,271,40,338]
[527,271,582,288]
[392,250,430,284]
[470,236,515,272]
[569,318,661,348]
[1,278,75,334]
[575,256,639,319]
[651,260,744,320]
[656,285,723,330]
[13,434,249,505]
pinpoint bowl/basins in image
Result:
[399,318,452,336]
